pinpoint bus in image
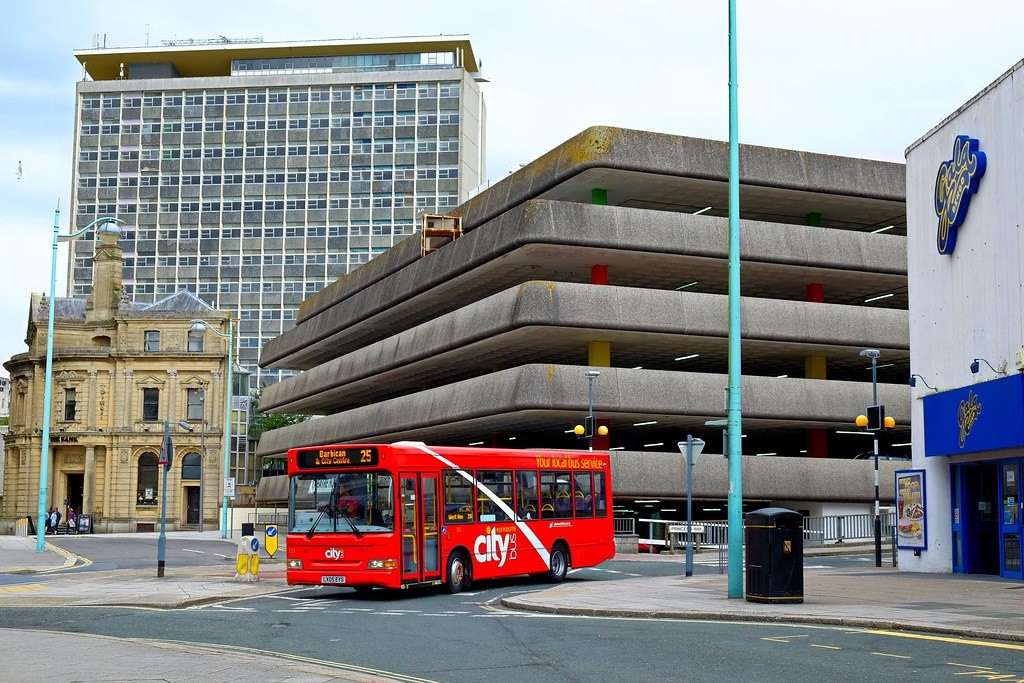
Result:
[286,440,616,592]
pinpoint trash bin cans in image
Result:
[744,505,805,605]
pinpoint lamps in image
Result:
[909,374,937,391]
[971,359,1007,376]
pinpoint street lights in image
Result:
[585,371,600,452]
[36,196,126,553]
[191,318,233,539]
[860,349,883,569]
[157,422,194,578]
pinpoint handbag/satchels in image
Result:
[69,519,76,527]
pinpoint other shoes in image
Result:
[76,531,78,535]
[51,533,54,535]
[55,531,57,535]
[65,533,69,535]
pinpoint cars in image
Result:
[615,529,656,553]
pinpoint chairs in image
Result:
[448,486,600,521]
[367,507,384,527]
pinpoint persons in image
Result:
[49,510,57,535]
[64,508,78,535]
[55,507,61,535]
[317,480,358,518]
[45,511,51,533]
